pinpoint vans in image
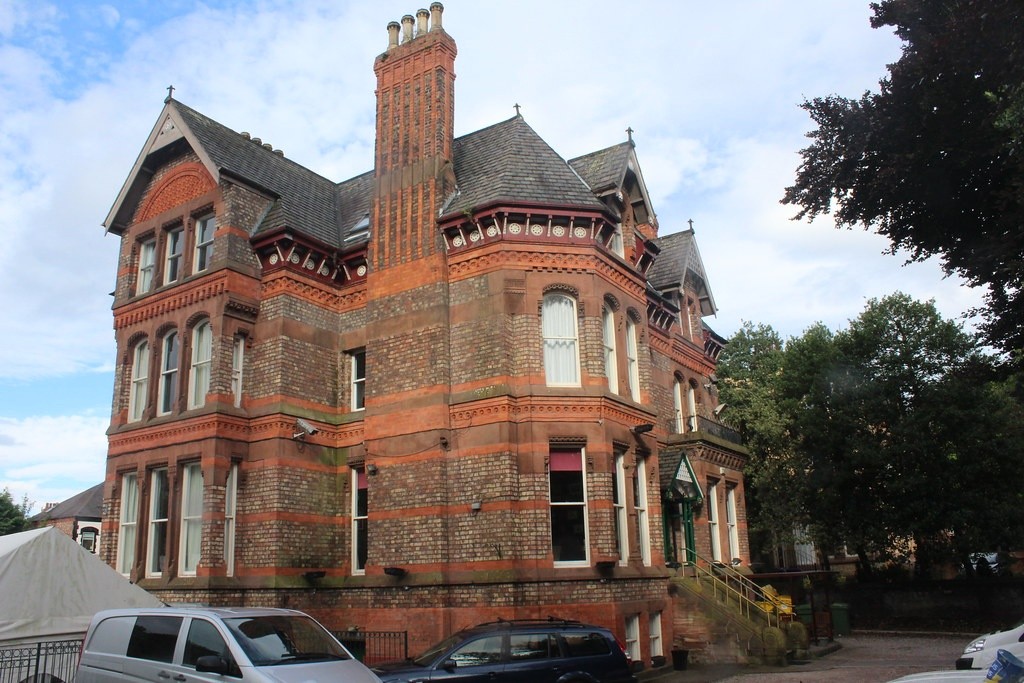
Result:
[73,606,383,683]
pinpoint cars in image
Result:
[885,667,1024,683]
[955,622,1024,669]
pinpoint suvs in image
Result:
[369,615,638,683]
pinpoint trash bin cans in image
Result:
[795,604,814,637]
[831,603,850,637]
